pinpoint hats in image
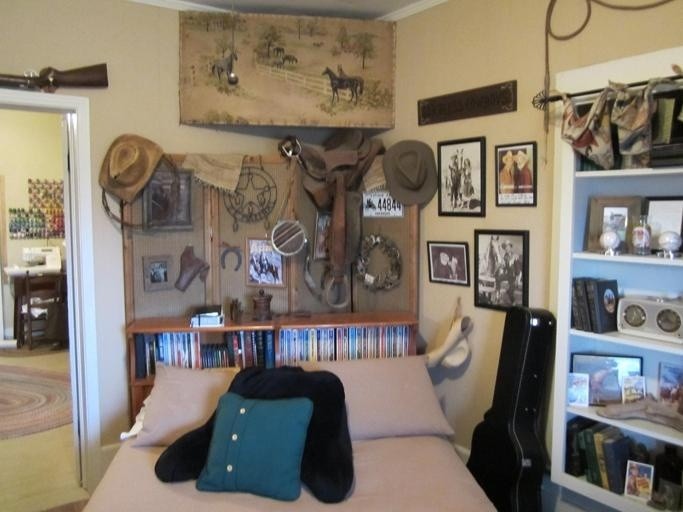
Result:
[382,139,440,207]
[97,132,165,205]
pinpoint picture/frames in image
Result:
[623,459,655,502]
[245,236,287,289]
[437,136,485,216]
[141,255,175,292]
[314,209,332,262]
[571,353,644,407]
[621,375,647,405]
[495,141,537,207]
[473,228,529,311]
[142,167,194,231]
[566,372,589,408]
[427,240,470,285]
[585,195,644,252]
[643,196,683,254]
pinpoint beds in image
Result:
[77,363,497,512]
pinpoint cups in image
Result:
[252,289,273,321]
[631,214,654,255]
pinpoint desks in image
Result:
[4,267,68,348]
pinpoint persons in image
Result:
[511,150,533,193]
[432,248,454,279]
[460,158,476,210]
[446,153,460,208]
[497,241,519,287]
[625,462,638,495]
[498,151,516,193]
[564,417,631,496]
[492,279,513,305]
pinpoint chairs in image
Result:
[22,270,65,351]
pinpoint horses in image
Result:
[282,55,297,65]
[322,67,358,106]
[337,64,363,95]
[212,51,238,82]
[273,47,285,57]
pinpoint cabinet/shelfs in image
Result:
[548,45,683,512]
[123,311,420,428]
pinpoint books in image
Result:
[570,276,617,333]
[125,324,414,380]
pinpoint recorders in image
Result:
[618,296,683,346]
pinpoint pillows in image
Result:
[294,356,454,440]
[197,393,312,501]
[132,360,243,447]
